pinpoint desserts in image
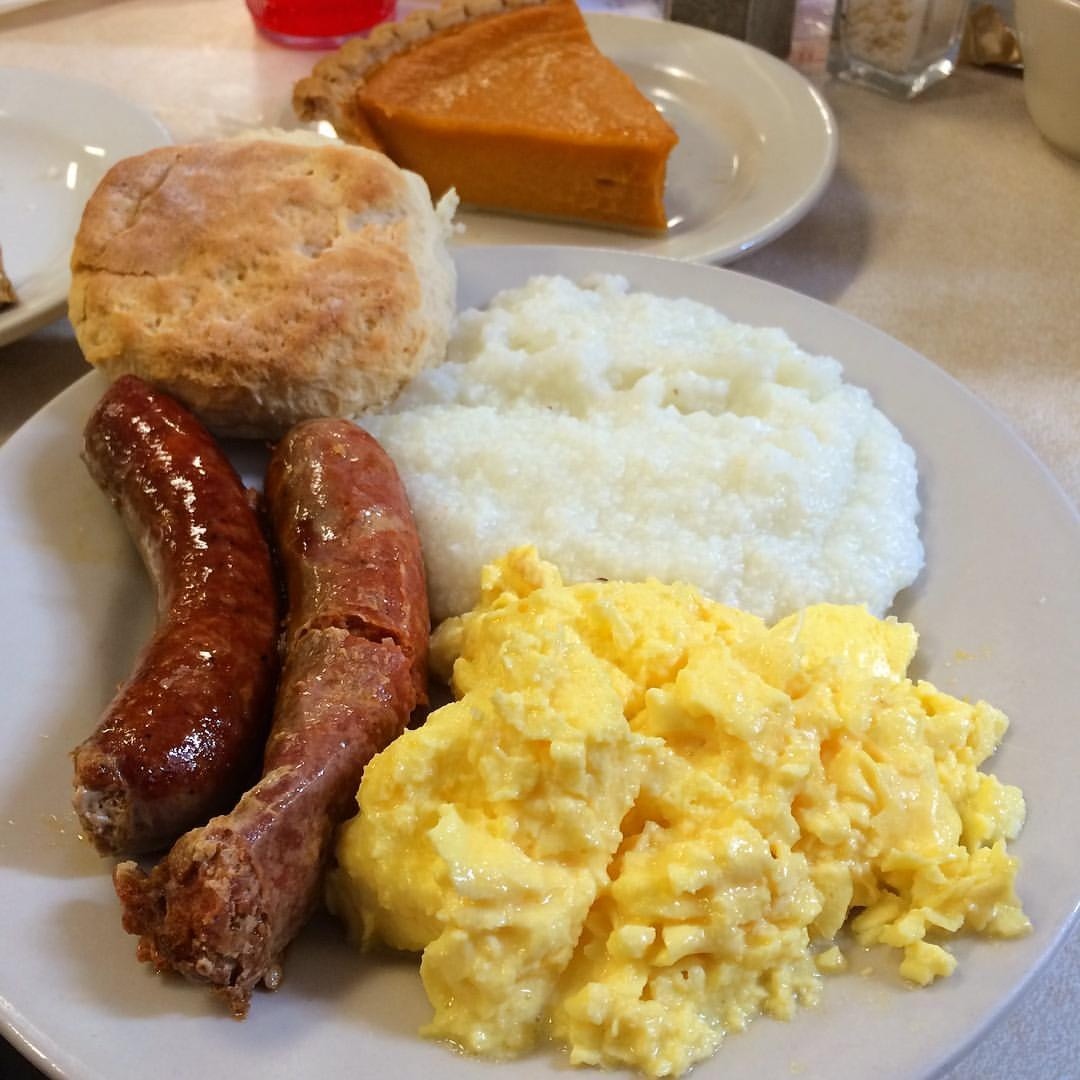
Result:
[295,0,675,240]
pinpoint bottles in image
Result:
[827,0,968,100]
[247,0,395,49]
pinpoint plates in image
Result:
[430,10,836,265]
[1,240,1077,1080]
[1,64,177,345]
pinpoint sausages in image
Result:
[64,371,430,1015]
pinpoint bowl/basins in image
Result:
[1013,1,1080,160]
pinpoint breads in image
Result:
[63,109,467,429]
